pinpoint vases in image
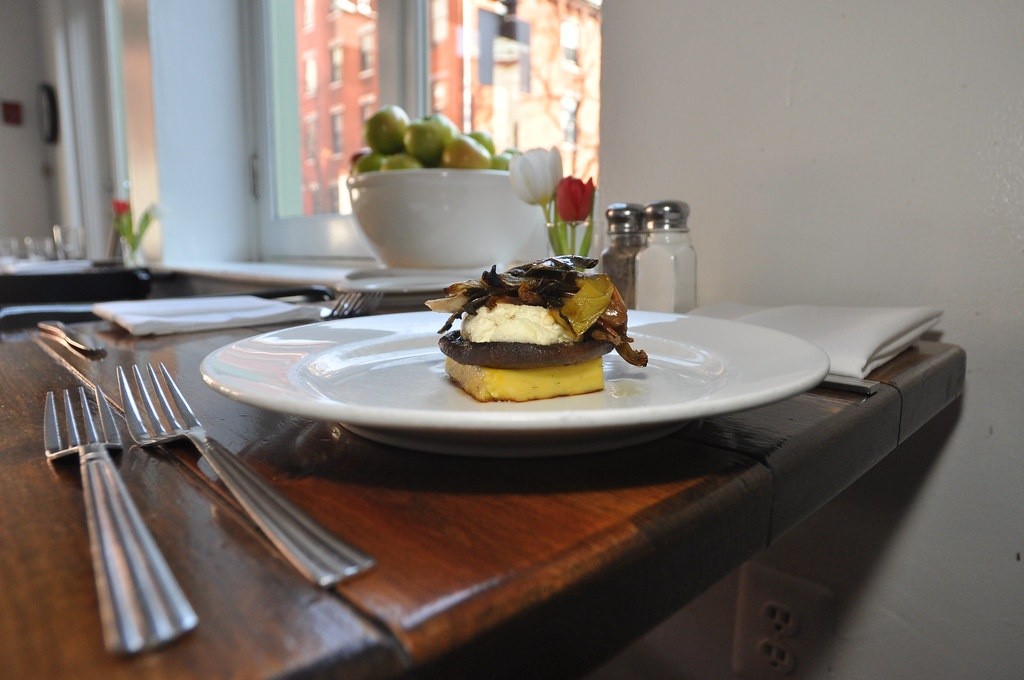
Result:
[544,220,601,275]
[120,237,146,271]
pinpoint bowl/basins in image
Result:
[345,168,535,278]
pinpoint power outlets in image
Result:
[730,558,836,680]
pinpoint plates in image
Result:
[200,310,833,456]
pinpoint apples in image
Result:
[349,103,521,173]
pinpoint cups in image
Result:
[0,225,82,262]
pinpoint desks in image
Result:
[0,263,154,332]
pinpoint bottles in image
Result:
[599,204,649,311]
[634,199,702,315]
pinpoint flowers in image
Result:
[508,144,595,224]
[110,198,155,251]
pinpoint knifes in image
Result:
[37,319,108,353]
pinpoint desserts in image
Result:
[437,302,614,403]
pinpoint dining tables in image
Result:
[0,326,967,680]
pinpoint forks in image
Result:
[117,360,377,588]
[330,290,386,318]
[44,383,199,657]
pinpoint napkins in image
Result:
[733,303,947,380]
[90,298,322,336]
